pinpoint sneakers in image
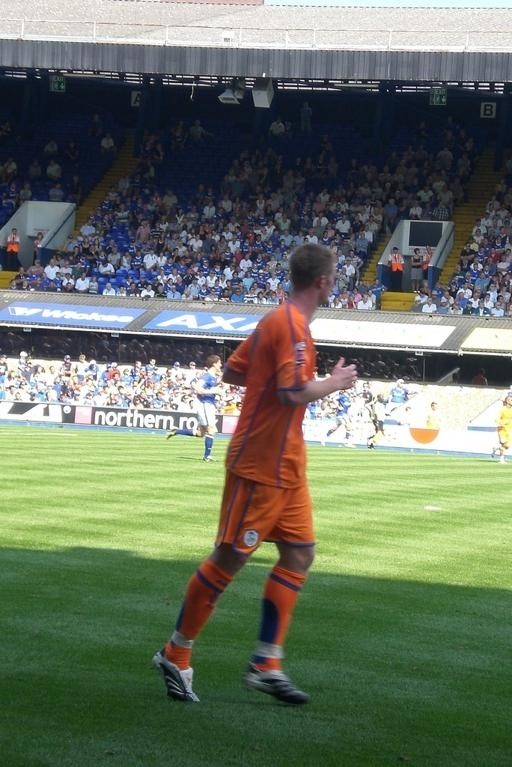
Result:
[202,456,215,463]
[152,648,200,702]
[242,662,311,705]
[166,426,178,440]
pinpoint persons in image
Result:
[152,242,358,705]
[321,389,355,448]
[490,396,512,465]
[165,354,223,463]
[367,394,386,450]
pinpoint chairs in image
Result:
[78,224,160,297]
